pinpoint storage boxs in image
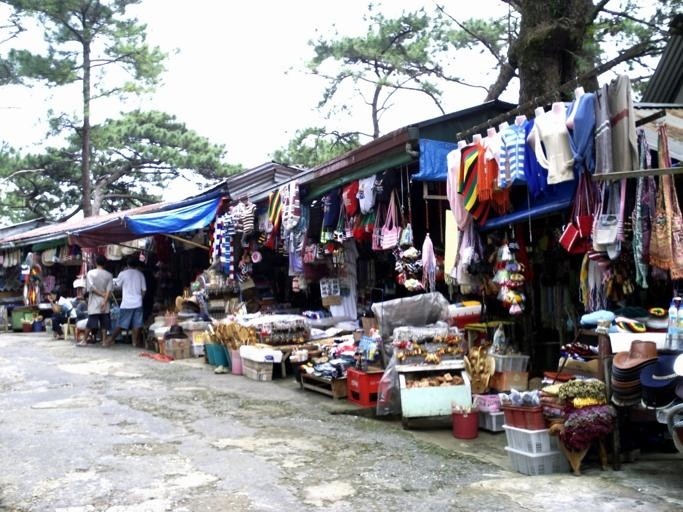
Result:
[500,401,571,476]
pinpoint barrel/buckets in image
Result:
[204,343,213,366]
[22,322,32,332]
[213,344,226,367]
[34,320,42,332]
[451,406,481,440]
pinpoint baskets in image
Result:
[503,446,570,476]
[501,424,560,454]
[472,394,500,413]
[498,405,549,430]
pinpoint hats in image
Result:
[165,326,187,339]
[610,340,682,410]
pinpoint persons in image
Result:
[447,84,598,226]
[74,291,101,343]
[46,289,78,340]
[73,254,113,348]
[101,256,147,348]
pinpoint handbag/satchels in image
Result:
[320,212,373,244]
[371,223,413,251]
[558,213,620,261]
